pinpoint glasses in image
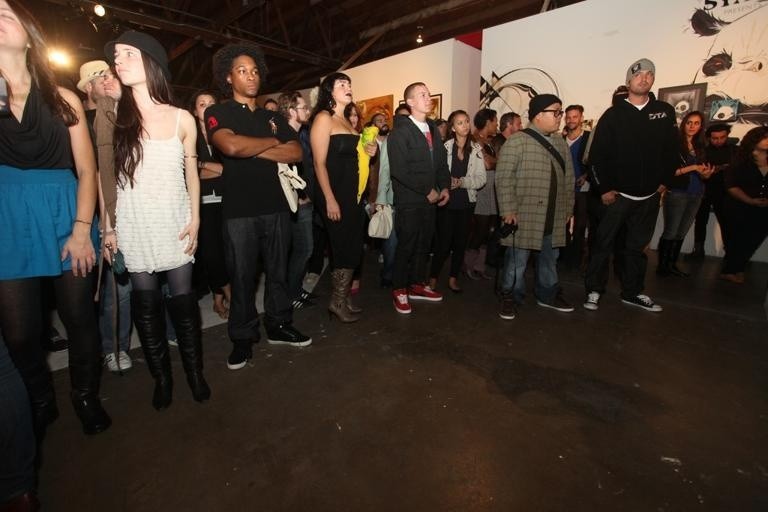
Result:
[540,110,564,117]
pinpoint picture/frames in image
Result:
[658,82,707,120]
[399,92,443,122]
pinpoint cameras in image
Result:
[498,218,518,239]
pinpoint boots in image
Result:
[657,236,689,282]
[327,268,363,323]
[166,292,211,403]
[128,290,173,410]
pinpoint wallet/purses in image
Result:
[106,243,126,273]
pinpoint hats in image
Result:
[77,60,110,93]
[104,29,170,81]
[529,94,562,121]
[626,58,655,83]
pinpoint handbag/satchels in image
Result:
[368,204,393,240]
[278,161,307,214]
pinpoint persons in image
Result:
[0,0,767,458]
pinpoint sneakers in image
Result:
[267,322,313,347]
[536,295,575,312]
[105,352,133,372]
[582,291,601,310]
[67,392,111,435]
[392,288,411,314]
[497,289,516,320]
[621,293,664,312]
[408,281,443,301]
[227,346,247,371]
